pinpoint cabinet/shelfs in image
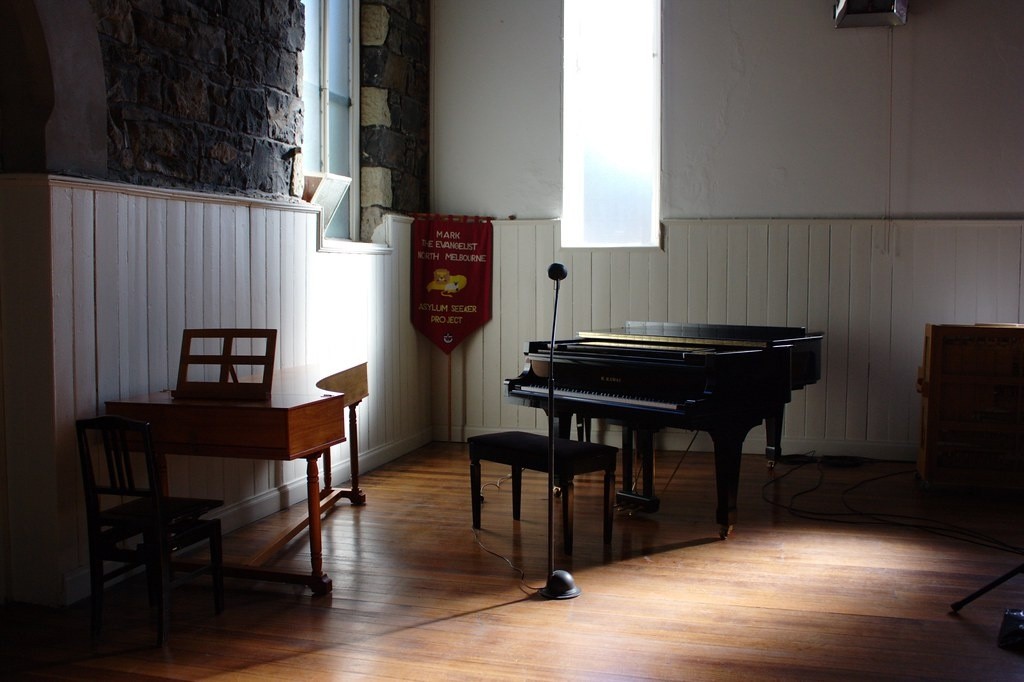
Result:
[914,324,1024,490]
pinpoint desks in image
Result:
[104,359,370,595]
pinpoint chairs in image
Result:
[74,417,227,647]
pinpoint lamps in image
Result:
[539,262,581,599]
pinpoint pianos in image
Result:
[99,355,367,601]
[500,316,829,541]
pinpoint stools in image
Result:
[465,430,618,556]
